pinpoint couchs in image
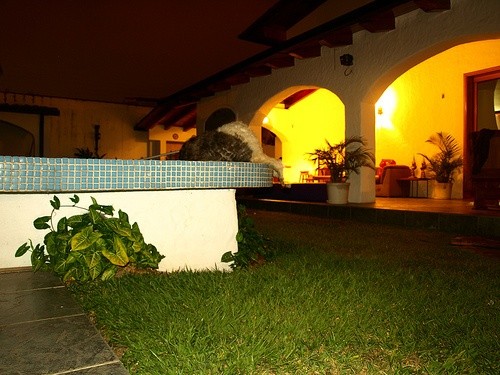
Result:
[376,165,413,197]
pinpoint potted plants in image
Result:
[416,131,465,199]
[307,136,377,203]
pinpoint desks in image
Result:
[311,175,332,183]
[399,176,436,198]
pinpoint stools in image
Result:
[299,171,309,183]
